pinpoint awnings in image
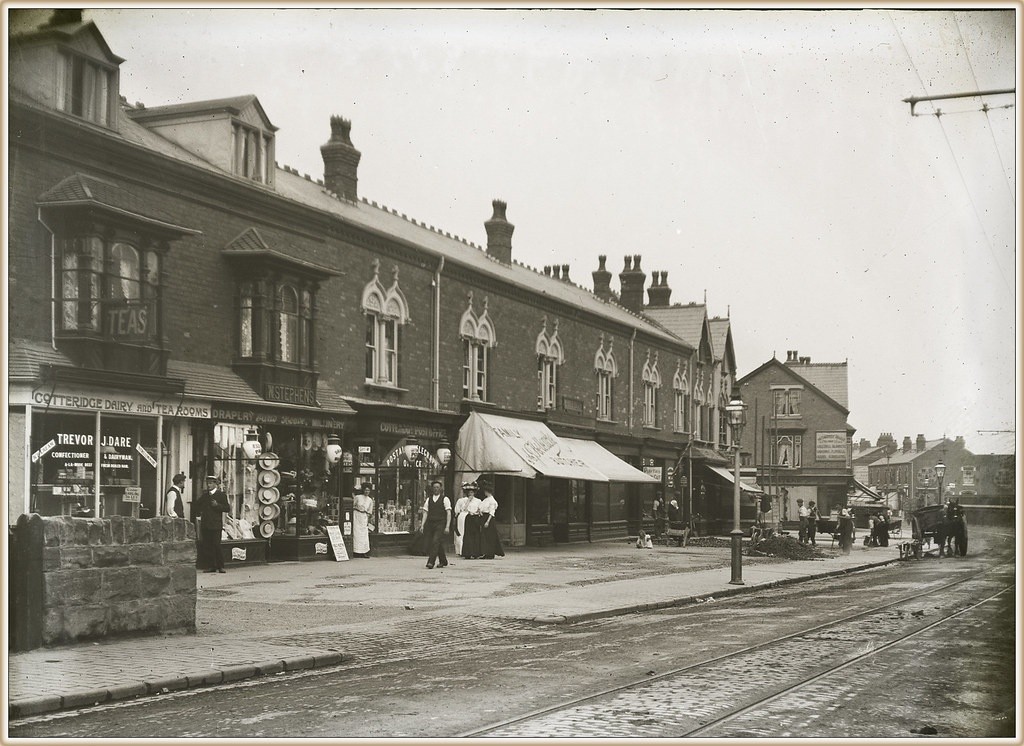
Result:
[453,409,663,505]
[705,463,763,492]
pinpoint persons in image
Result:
[797,499,910,549]
[410,479,505,559]
[165,475,186,519]
[194,476,230,574]
[420,481,453,568]
[942,487,956,518]
[620,496,626,518]
[353,483,373,558]
[636,491,681,549]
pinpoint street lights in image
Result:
[723,394,749,585]
[935,459,948,505]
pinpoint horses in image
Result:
[937,499,968,558]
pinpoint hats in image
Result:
[461,481,480,490]
[205,476,217,481]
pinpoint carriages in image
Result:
[911,498,968,559]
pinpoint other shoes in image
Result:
[354,553,370,559]
[426,563,434,569]
[437,560,448,568]
[219,569,227,573]
[203,569,216,573]
